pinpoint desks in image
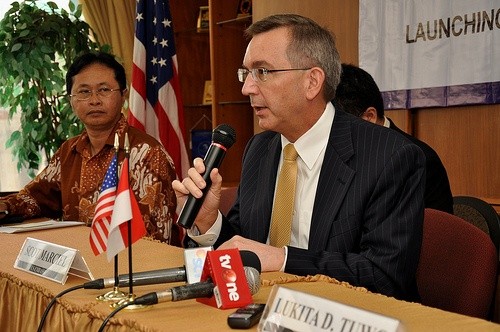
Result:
[0,218,500,332]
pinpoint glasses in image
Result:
[236,66,312,83]
[69,86,122,101]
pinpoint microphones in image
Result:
[83,246,261,290]
[176,124,236,229]
[133,248,261,309]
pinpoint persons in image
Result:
[334,64,454,215]
[172,14,425,302]
[0,52,176,244]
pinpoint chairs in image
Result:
[421,193,500,318]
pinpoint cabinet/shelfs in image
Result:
[171,0,252,185]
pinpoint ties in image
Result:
[269,144,298,248]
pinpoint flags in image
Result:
[128,0,194,246]
[89,152,146,262]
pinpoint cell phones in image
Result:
[228,302,266,329]
